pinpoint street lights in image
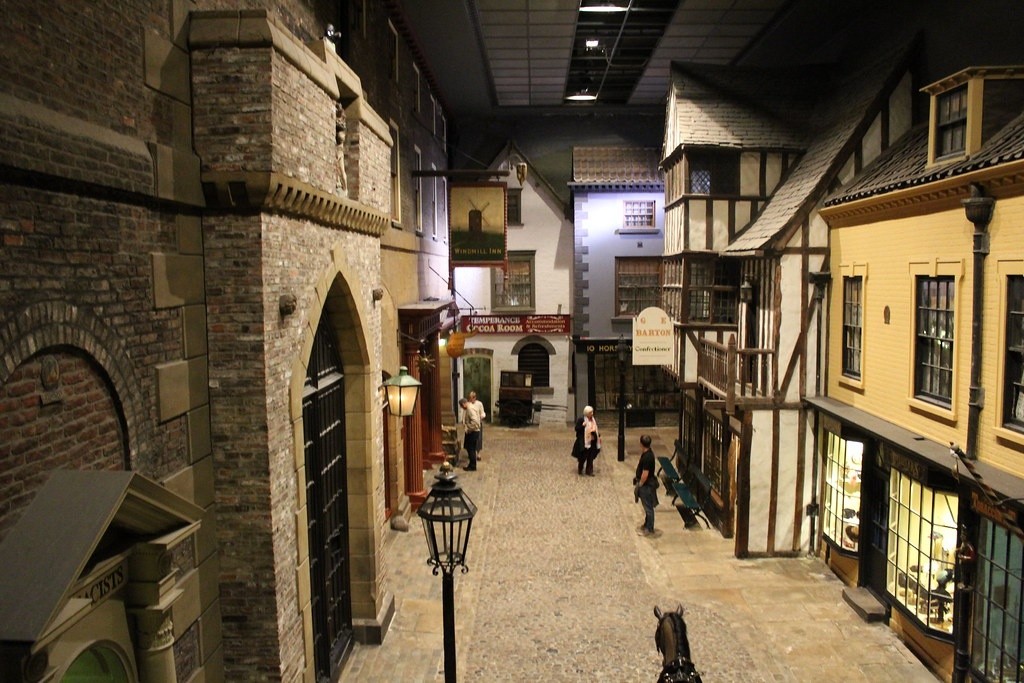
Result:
[615,333,631,461]
[416,461,478,683]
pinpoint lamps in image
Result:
[565,78,597,100]
[740,272,753,303]
[380,365,422,419]
[507,153,527,187]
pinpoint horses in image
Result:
[654,603,702,683]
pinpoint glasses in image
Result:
[469,395,476,399]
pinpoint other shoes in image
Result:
[578,472,596,477]
[635,523,655,536]
[463,455,482,471]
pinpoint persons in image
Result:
[635,434,658,535]
[574,406,602,477]
[462,391,487,460]
[459,398,481,470]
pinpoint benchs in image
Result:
[671,462,713,530]
[656,439,691,496]
